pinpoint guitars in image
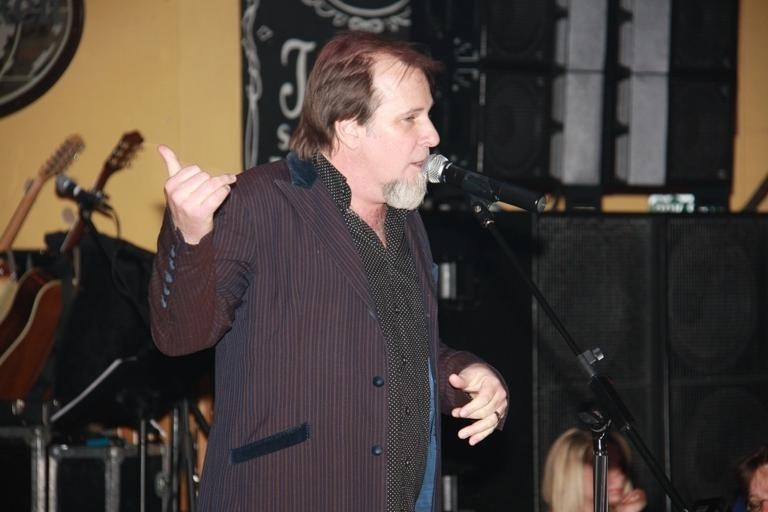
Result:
[0,131,143,402]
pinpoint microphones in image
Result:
[55,176,114,212]
[420,153,547,216]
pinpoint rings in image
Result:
[494,410,502,424]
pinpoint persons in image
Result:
[542,425,646,511]
[739,448,768,511]
[146,31,514,511]
